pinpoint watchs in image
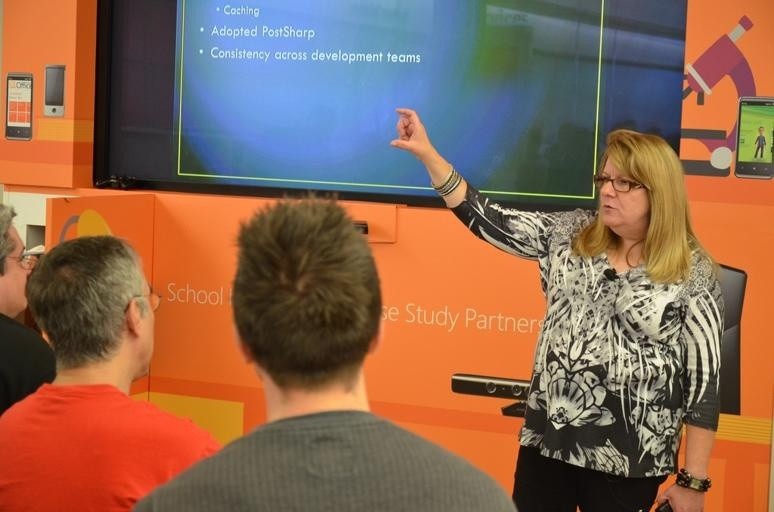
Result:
[674,468,711,493]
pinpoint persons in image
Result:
[0,201,58,414]
[127,186,524,512]
[1,233,224,512]
[390,107,725,512]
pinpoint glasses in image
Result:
[11,255,42,269]
[123,292,161,316]
[594,171,644,193]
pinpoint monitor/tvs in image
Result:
[92,0,689,213]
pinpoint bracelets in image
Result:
[431,164,463,198]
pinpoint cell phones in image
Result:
[5,72,33,141]
[43,64,66,117]
[734,96,773,180]
